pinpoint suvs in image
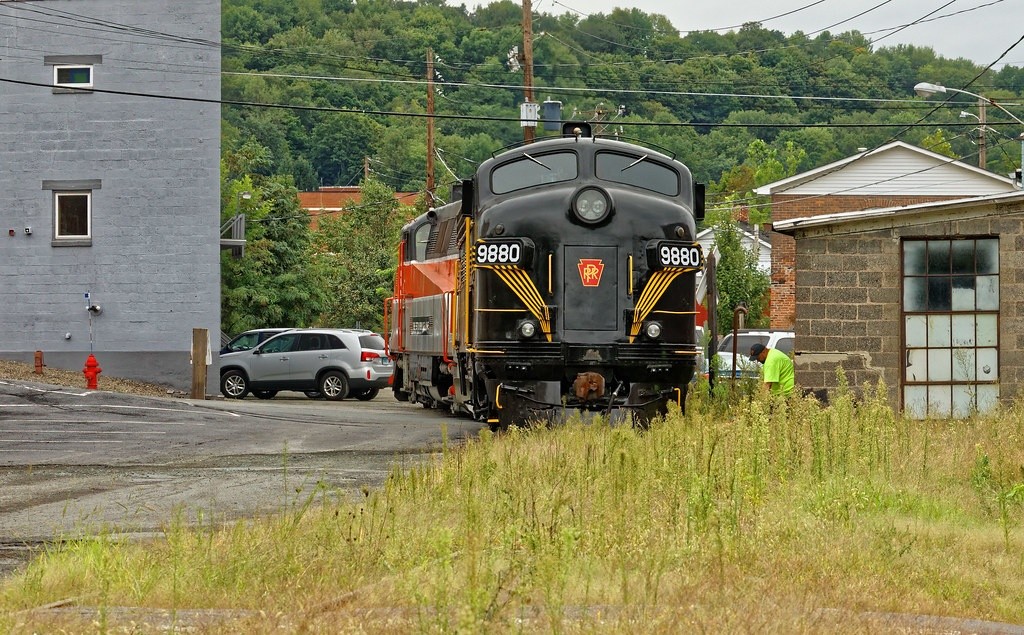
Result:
[219,327,394,404]
[709,330,796,375]
[221,327,334,400]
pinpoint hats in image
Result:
[749,344,765,361]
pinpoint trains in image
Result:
[382,122,710,434]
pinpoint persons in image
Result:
[749,344,794,399]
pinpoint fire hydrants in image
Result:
[82,355,102,389]
